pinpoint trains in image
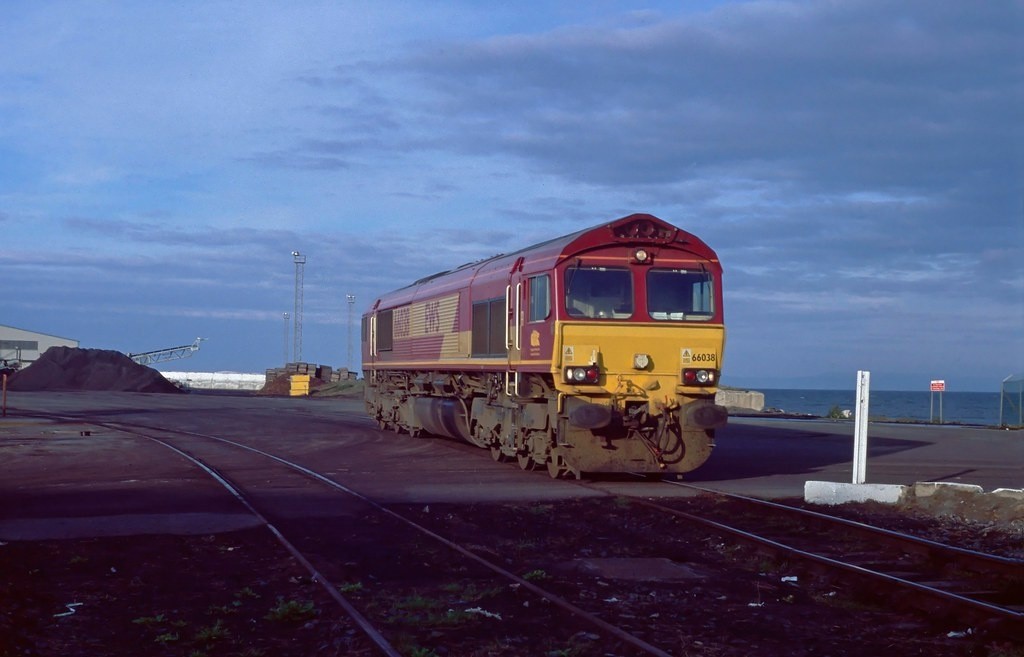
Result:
[362,213,729,483]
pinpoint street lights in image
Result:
[291,251,306,363]
[282,312,290,366]
[347,295,356,372]
